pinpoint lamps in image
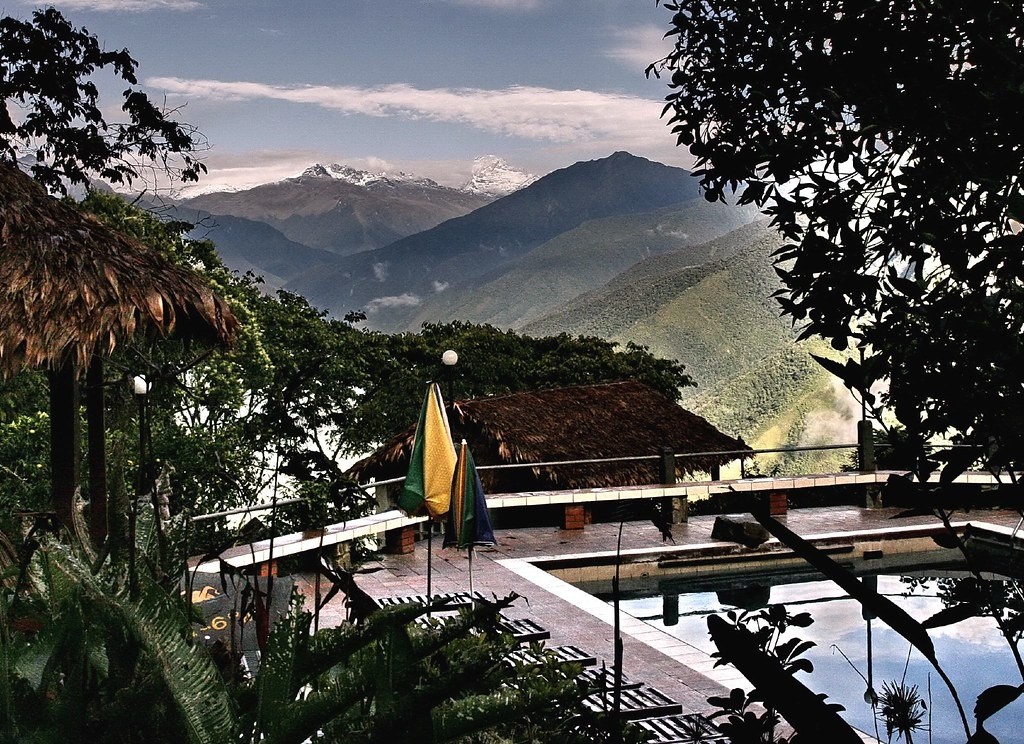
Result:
[135,377,153,393]
[442,350,459,366]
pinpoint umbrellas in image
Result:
[442,438,500,613]
[398,379,458,617]
